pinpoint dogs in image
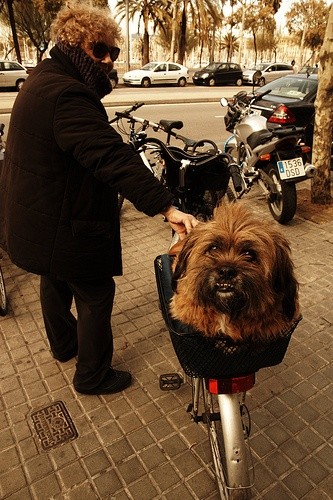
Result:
[169,196,301,342]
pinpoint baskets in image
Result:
[153,251,306,380]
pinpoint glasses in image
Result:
[88,38,122,63]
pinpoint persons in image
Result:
[0,3,206,394]
[291,60,295,70]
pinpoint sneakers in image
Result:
[50,340,79,364]
[73,366,134,397]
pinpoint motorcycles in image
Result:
[219,69,318,225]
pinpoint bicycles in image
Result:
[109,101,237,220]
[0,123,7,317]
[131,131,303,500]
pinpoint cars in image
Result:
[0,60,29,92]
[247,73,318,150]
[122,61,190,88]
[192,60,244,87]
[242,62,296,87]
